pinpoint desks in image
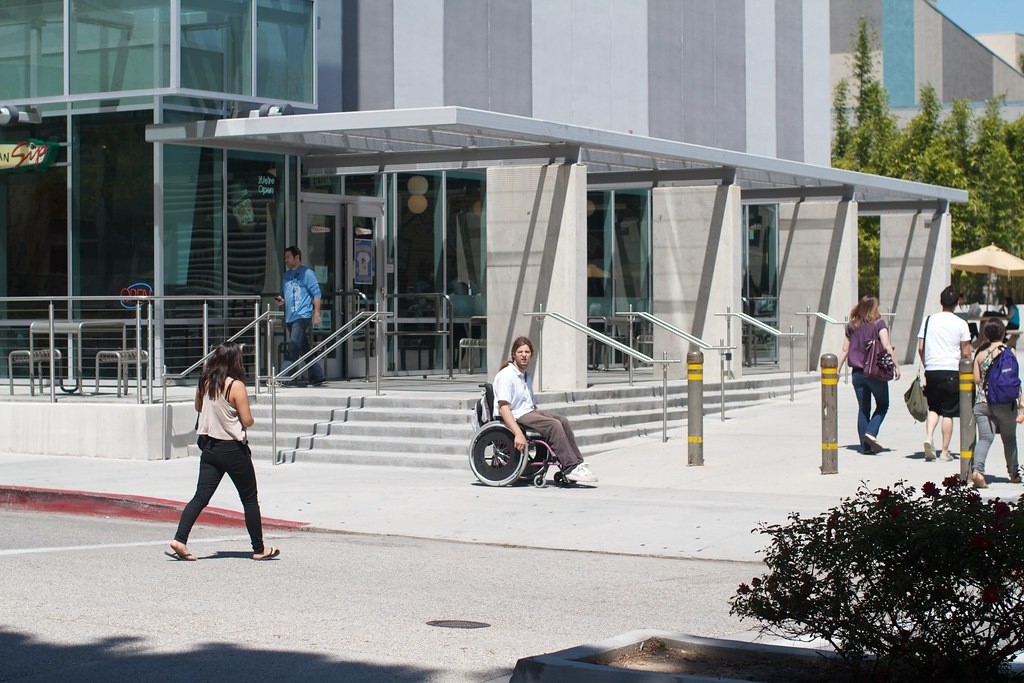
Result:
[468,315,487,375]
[28,319,127,395]
[587,315,642,370]
[968,317,1012,349]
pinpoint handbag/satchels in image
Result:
[904,378,928,422]
[862,320,893,381]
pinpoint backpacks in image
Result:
[983,345,1022,404]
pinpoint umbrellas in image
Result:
[951,242,1024,311]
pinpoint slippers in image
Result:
[257,548,279,560]
[164,551,194,561]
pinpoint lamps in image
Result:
[406,176,430,214]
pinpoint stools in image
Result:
[94,351,149,398]
[8,347,64,395]
[460,337,486,374]
[588,335,654,368]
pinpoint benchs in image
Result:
[1006,330,1024,335]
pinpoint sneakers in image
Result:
[566,462,598,482]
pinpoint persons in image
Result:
[164,342,280,561]
[917,287,971,461]
[1002,297,1020,344]
[275,246,328,387]
[836,295,900,454]
[954,294,979,343]
[396,255,481,370]
[971,318,1024,488]
[494,339,598,482]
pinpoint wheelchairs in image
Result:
[469,383,584,488]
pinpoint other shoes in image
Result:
[281,381,297,388]
[924,441,937,460]
[971,474,987,488]
[863,448,873,453]
[863,435,883,451]
[940,453,953,461]
[307,379,328,387]
[1011,476,1021,483]
